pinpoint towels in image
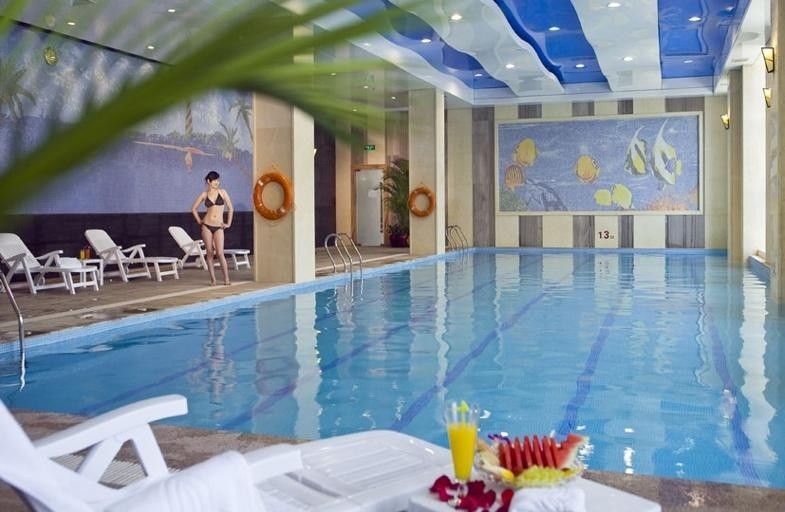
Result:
[102,451,266,512]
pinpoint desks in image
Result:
[410,466,662,511]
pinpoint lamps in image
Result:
[721,113,729,129]
[763,88,771,108]
[761,47,774,73]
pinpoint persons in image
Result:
[190,170,234,287]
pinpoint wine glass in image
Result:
[444,398,482,508]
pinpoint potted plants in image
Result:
[374,159,408,248]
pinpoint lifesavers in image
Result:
[253,172,292,221]
[409,187,435,217]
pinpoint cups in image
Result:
[79,248,91,260]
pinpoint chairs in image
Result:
[0,226,251,295]
[1,393,454,511]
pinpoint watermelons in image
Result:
[499,433,589,476]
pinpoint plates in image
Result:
[471,447,585,492]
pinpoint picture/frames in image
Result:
[494,112,703,216]
